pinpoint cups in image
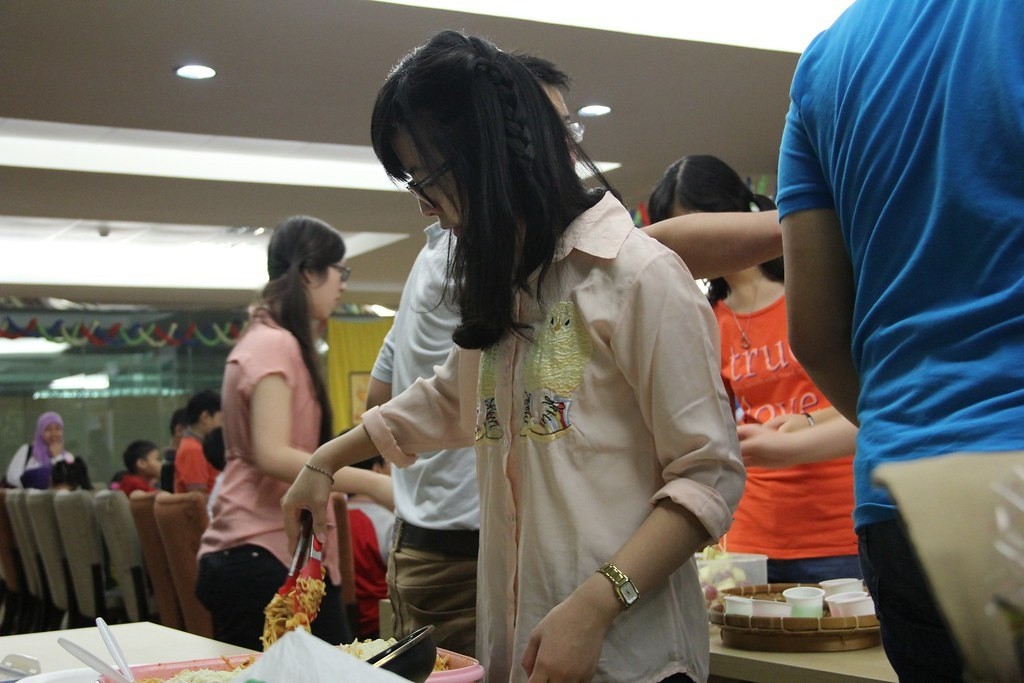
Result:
[723,595,752,616]
[823,579,864,606]
[752,600,793,617]
[819,578,858,589]
[825,591,868,619]
[782,586,825,619]
[834,596,875,618]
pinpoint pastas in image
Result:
[221,578,326,670]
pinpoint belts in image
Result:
[399,521,480,554]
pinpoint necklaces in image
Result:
[727,270,764,348]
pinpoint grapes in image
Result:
[700,571,749,611]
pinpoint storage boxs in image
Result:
[696,553,769,603]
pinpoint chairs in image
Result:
[0,488,365,644]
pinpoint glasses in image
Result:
[328,263,351,282]
[566,121,584,144]
[404,161,452,210]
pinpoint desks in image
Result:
[706,619,900,683]
[0,618,265,683]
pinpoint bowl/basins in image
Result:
[364,623,437,683]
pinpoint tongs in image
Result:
[262,512,322,639]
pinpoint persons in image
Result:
[774,0,1023,681]
[643,153,863,583]
[6,391,397,640]
[364,50,577,656]
[189,215,394,654]
[279,28,746,683]
[365,49,782,656]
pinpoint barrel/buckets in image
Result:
[695,553,768,612]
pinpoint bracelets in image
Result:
[305,462,334,483]
[801,411,816,426]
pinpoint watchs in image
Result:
[591,561,640,609]
